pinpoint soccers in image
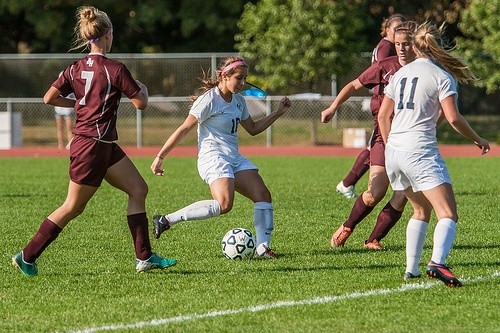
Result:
[221,228,256,260]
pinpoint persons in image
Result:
[321,13,490,287]
[54,92,74,149]
[12,5,177,278]
[150,56,292,258]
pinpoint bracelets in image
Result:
[156,156,164,159]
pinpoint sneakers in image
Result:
[331,224,352,248]
[404,271,424,280]
[425,260,463,288]
[13,250,41,278]
[364,239,387,251]
[134,253,177,273]
[152,214,171,239]
[252,245,282,260]
[335,181,356,199]
